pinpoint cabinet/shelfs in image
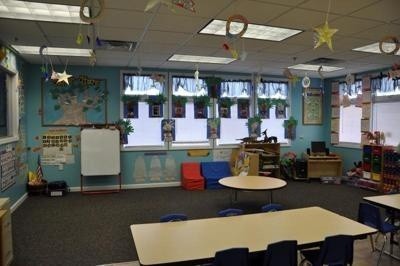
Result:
[232,141,281,183]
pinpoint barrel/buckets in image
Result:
[27,179,47,196]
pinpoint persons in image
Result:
[280,151,297,181]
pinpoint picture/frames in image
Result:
[301,86,326,125]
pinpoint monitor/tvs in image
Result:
[311,141,325,152]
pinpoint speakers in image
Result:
[307,148,310,156]
[326,148,329,156]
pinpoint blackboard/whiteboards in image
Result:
[80,123,121,176]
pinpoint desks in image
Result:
[302,148,343,182]
[216,176,289,207]
[362,192,400,211]
[129,204,379,265]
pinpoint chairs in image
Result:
[262,204,284,212]
[212,247,252,265]
[384,190,400,225]
[182,161,233,192]
[357,201,399,265]
[217,208,244,216]
[265,241,300,265]
[302,235,355,265]
[158,213,186,222]
